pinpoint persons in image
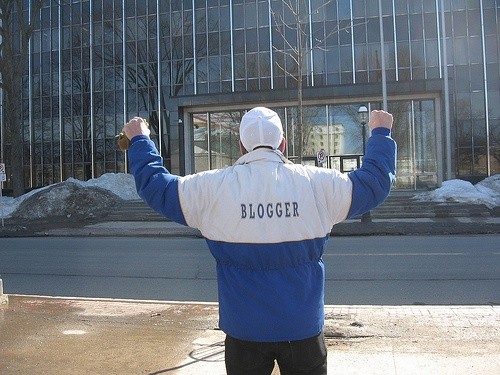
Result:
[123,106,397,375]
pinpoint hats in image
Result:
[240,107,283,153]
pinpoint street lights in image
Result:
[357,104,373,223]
